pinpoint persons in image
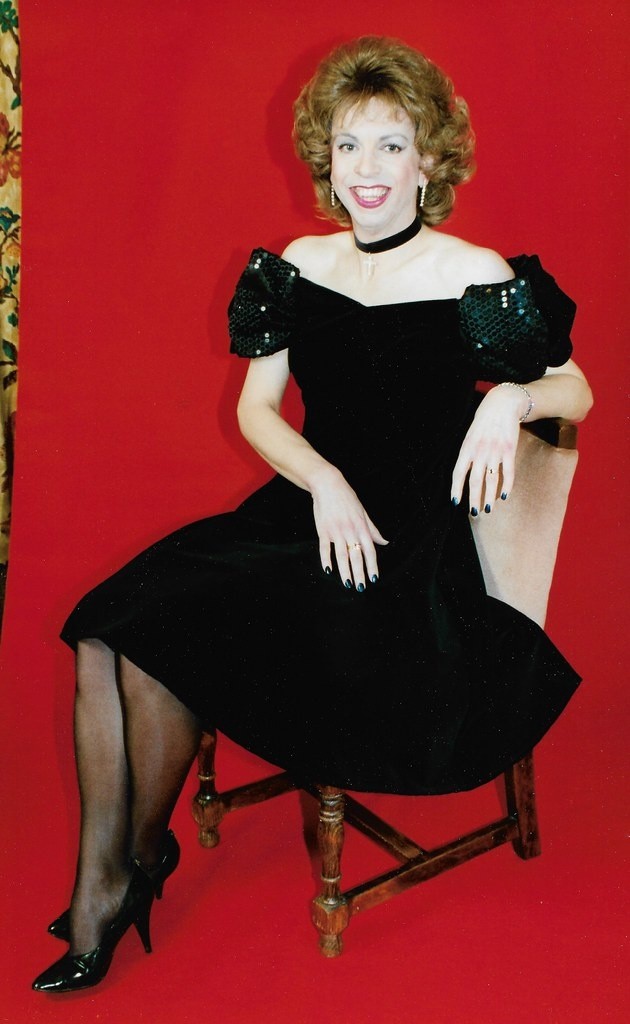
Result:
[32,34,593,993]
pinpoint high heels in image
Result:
[29,827,182,992]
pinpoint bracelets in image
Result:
[498,382,532,423]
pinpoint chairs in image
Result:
[192,392,578,957]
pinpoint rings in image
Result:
[488,469,498,473]
[348,544,361,550]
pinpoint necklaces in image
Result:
[354,214,422,275]
